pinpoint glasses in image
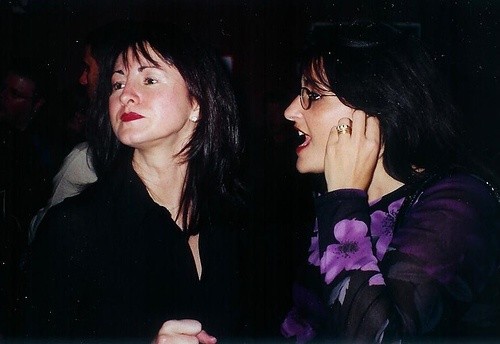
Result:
[295,80,337,110]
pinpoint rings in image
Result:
[336,123,352,134]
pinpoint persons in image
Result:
[31,21,295,344]
[0,33,118,245]
[277,17,500,344]
